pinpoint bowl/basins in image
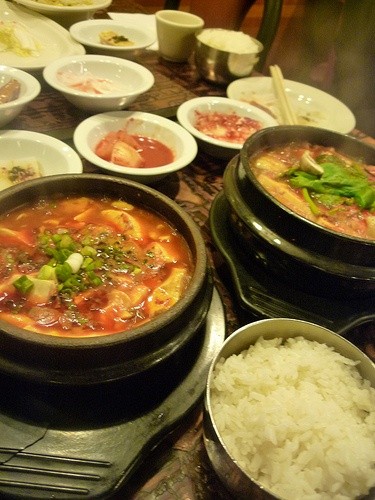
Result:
[0,65,41,127]
[193,27,264,89]
[0,174,207,386]
[176,96,278,161]
[72,111,198,185]
[203,316,375,500]
[43,53,155,115]
[238,124,375,269]
[0,130,83,191]
[14,0,113,30]
[222,152,375,301]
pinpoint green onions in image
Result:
[7,232,157,325]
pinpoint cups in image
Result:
[155,10,205,63]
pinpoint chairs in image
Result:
[163,0,284,73]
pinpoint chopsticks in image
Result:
[270,64,297,125]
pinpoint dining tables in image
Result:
[0,0,375,500]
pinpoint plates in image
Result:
[226,77,356,134]
[70,19,157,59]
[0,0,86,73]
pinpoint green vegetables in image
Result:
[277,152,375,218]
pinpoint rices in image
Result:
[210,337,375,500]
